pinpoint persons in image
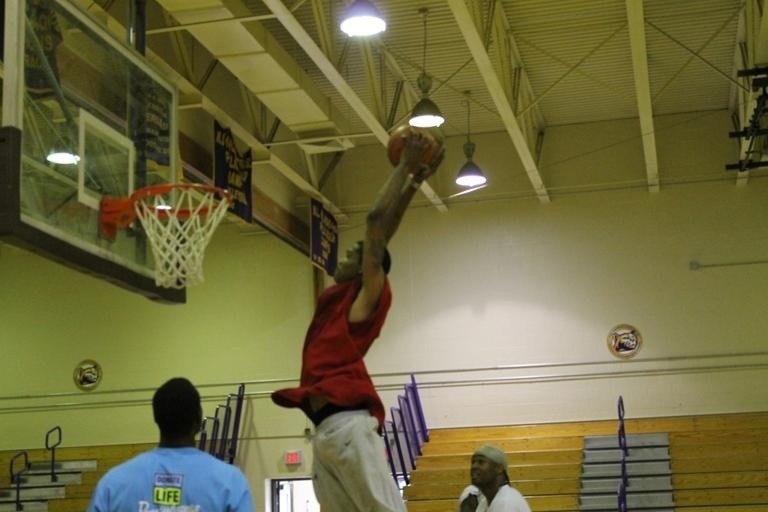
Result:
[269,126,445,512]
[88,377,256,511]
[458,444,531,512]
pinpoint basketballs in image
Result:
[387,124,441,173]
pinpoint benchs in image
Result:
[1,440,230,512]
[403,411,768,512]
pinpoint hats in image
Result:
[474,445,510,485]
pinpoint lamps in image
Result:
[408,9,446,131]
[340,1,386,40]
[44,124,81,165]
[453,92,487,188]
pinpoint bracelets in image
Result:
[410,181,422,190]
